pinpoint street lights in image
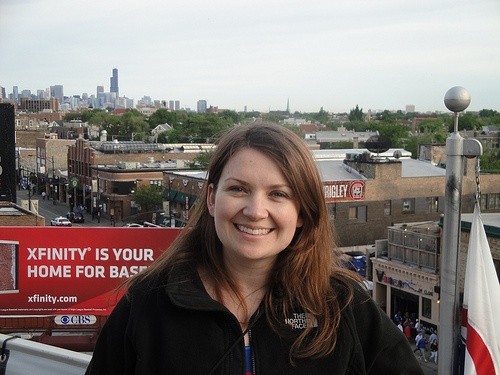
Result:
[167,176,174,226]
[23,168,31,211]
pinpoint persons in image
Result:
[84,121,424,375]
[393,311,438,363]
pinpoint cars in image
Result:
[67,211,84,223]
[50,216,72,227]
[122,224,144,228]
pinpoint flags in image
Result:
[459,155,500,375]
